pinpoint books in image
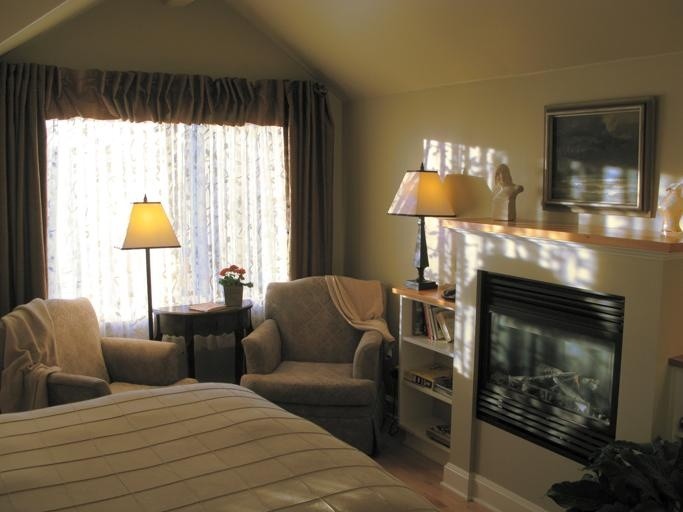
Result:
[411,300,453,343]
[404,364,453,398]
[426,424,450,449]
[188,304,224,313]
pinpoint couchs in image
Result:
[0,298,200,406]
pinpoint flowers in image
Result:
[219,265,253,288]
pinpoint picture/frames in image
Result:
[541,94,656,219]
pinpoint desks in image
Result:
[153,300,253,384]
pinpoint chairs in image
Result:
[241,275,386,456]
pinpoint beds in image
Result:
[0,383,442,512]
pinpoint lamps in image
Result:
[121,193,181,339]
[386,161,456,291]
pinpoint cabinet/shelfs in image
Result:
[390,286,456,466]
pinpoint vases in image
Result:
[224,284,243,306]
